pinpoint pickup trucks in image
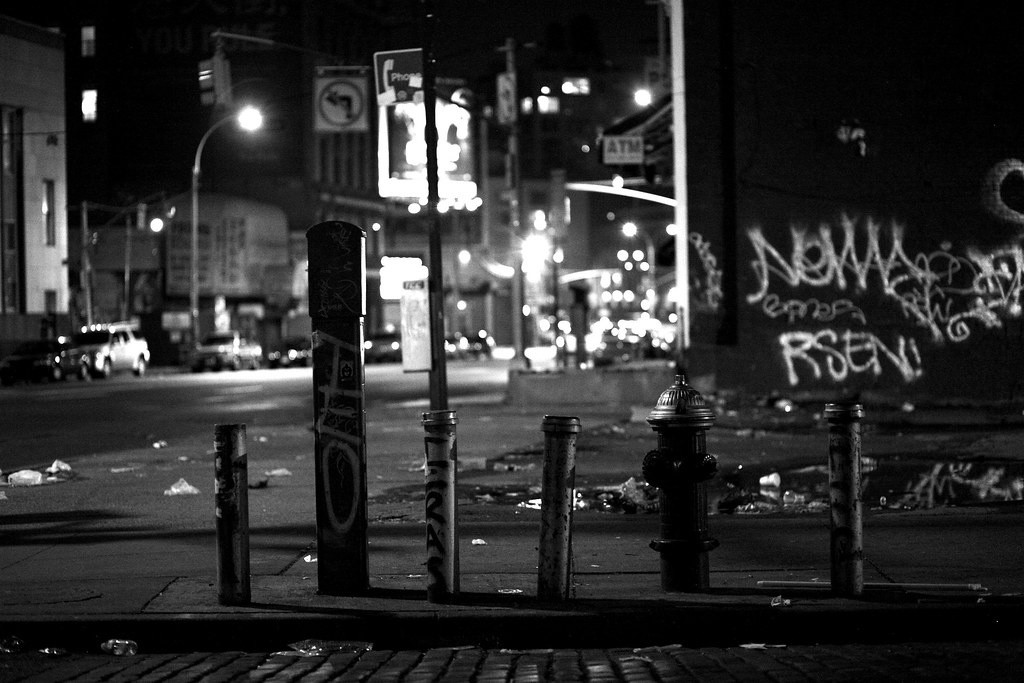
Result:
[190,330,262,374]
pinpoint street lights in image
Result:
[190,105,261,350]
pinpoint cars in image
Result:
[266,336,314,368]
[364,334,403,361]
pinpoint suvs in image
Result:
[53,323,152,382]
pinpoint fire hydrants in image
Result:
[642,370,720,597]
